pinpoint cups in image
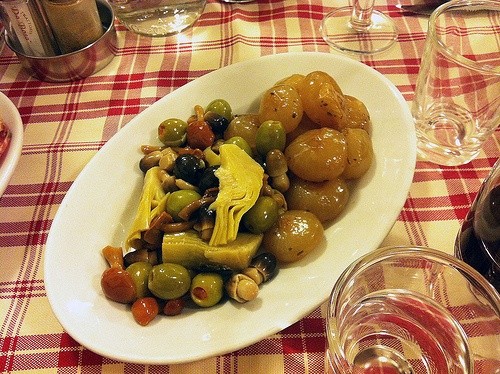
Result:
[454,157,500,295]
[412,0,500,167]
[325,244,500,374]
[111,0,207,35]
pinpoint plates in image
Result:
[42,51,418,366]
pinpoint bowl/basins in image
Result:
[3,0,117,84]
[0,92,24,198]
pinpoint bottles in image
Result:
[41,0,105,55]
[0,0,61,57]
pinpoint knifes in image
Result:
[402,2,447,15]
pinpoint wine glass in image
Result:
[319,0,400,57]
